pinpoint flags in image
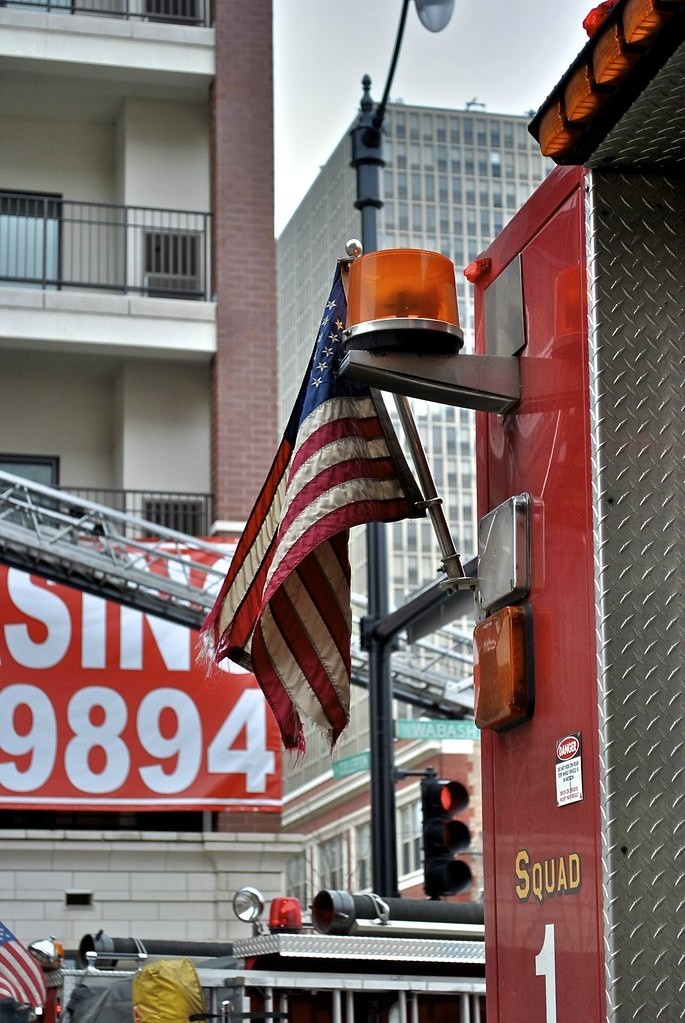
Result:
[190,254,431,769]
[0,919,48,1010]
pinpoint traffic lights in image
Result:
[417,770,475,896]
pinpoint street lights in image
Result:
[350,2,454,893]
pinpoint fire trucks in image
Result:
[0,469,485,1023]
[341,1,685,1023]
